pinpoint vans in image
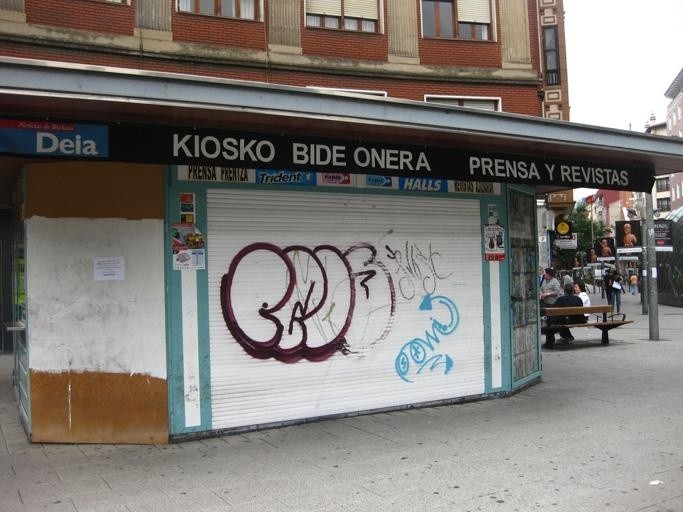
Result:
[553,261,616,286]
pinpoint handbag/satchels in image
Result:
[612,280,622,290]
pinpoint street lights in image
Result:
[626,207,660,315]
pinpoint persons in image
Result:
[541,268,639,348]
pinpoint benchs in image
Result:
[542,306,633,346]
